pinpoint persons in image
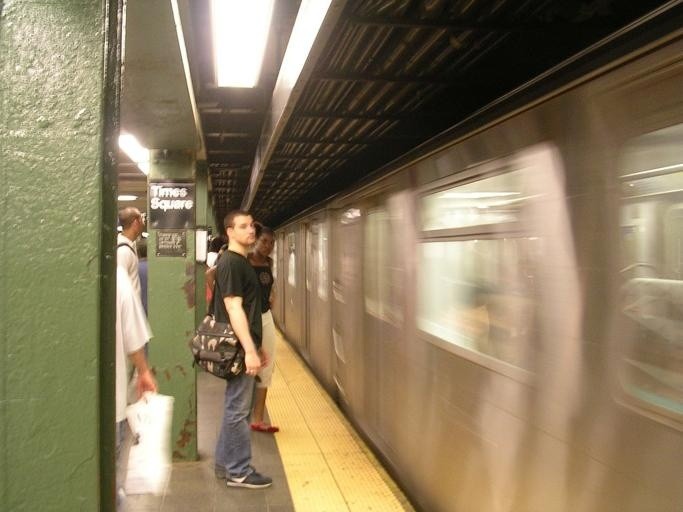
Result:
[205,209,282,490]
[117,206,145,388]
[117,264,157,469]
[135,240,147,315]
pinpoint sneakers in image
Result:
[226,470,274,489]
[216,464,256,479]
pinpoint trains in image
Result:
[260,2,681,510]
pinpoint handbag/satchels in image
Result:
[124,391,176,495]
[189,316,245,380]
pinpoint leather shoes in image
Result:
[250,424,279,433]
